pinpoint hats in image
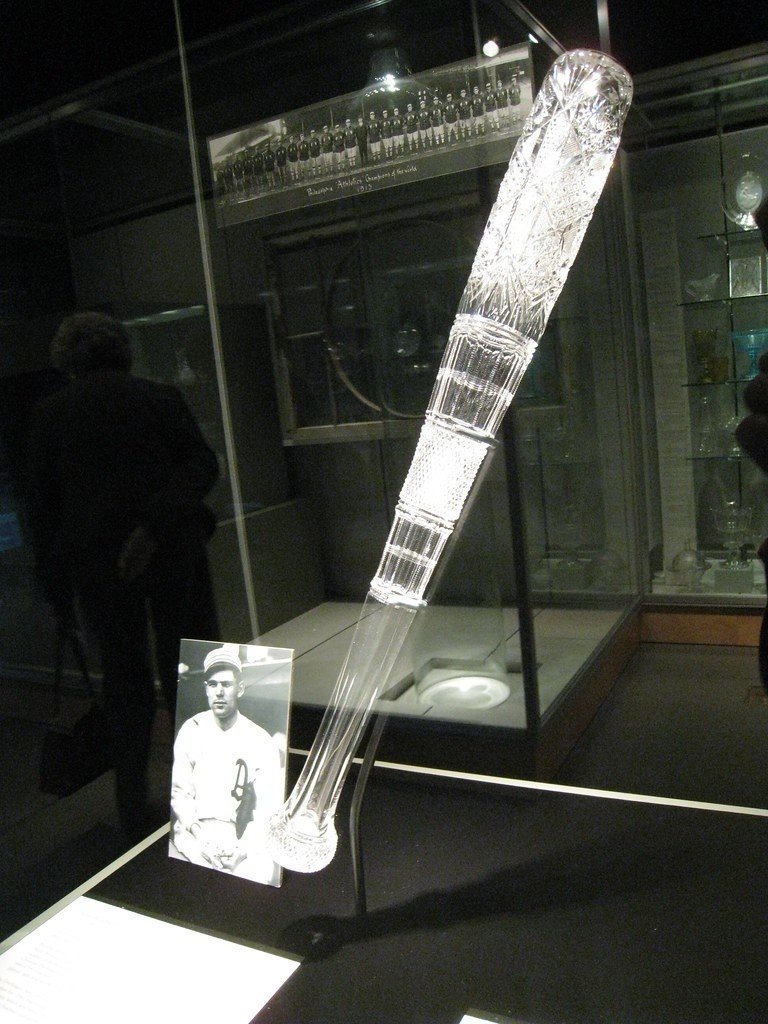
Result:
[202,646,242,680]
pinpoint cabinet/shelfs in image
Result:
[625,124,768,649]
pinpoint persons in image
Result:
[15,315,221,844]
[171,644,282,886]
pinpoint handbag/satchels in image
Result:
[38,621,107,796]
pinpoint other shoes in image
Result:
[116,777,149,844]
